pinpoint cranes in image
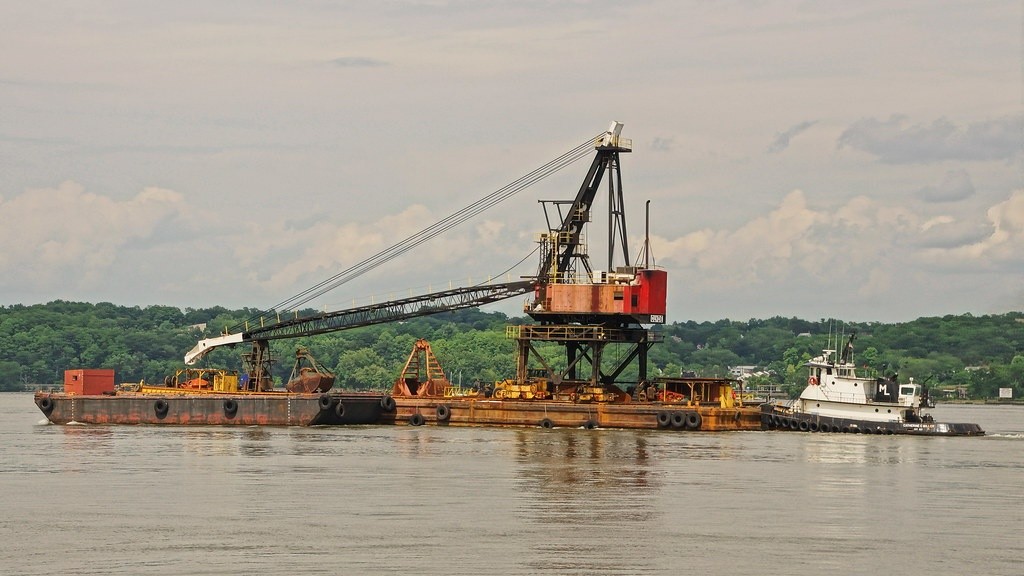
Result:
[178,120,669,405]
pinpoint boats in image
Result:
[387,339,763,433]
[34,364,398,431]
[763,318,990,437]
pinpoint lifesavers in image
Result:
[410,414,424,426]
[809,376,817,385]
[318,394,334,411]
[335,403,346,418]
[540,418,553,429]
[686,412,702,428]
[735,411,742,422]
[584,419,598,429]
[223,400,238,413]
[435,404,451,420]
[656,410,670,426]
[39,397,52,411]
[380,396,395,411]
[765,413,896,436]
[670,410,685,428]
[154,399,169,414]
